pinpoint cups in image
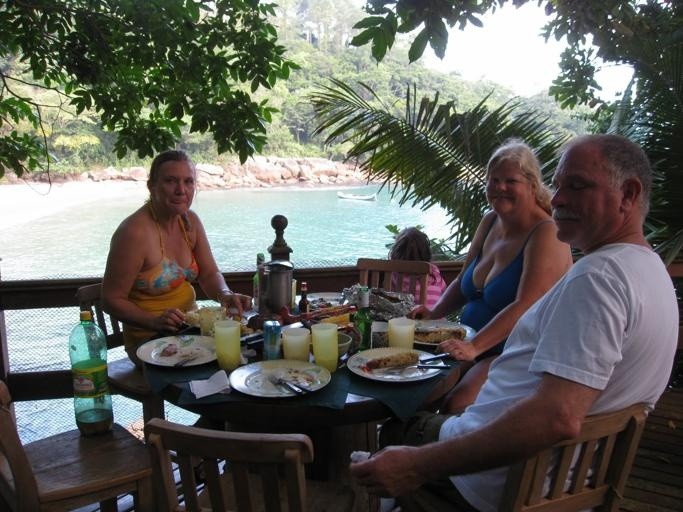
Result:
[214,320,240,373]
[387,318,414,348]
[310,323,337,373]
[281,328,309,361]
[291,279,296,309]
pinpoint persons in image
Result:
[347,133,679,511]
[386,227,449,320]
[101,150,253,484]
[396,145,574,414]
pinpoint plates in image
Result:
[348,321,388,333]
[413,318,477,346]
[181,307,259,328]
[346,348,444,383]
[135,334,216,367]
[228,358,331,397]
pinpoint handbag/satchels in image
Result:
[343,283,415,320]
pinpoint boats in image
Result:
[335,191,376,200]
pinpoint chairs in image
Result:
[356,258,430,306]
[144,418,354,510]
[497,403,646,511]
[76,283,179,512]
[0,380,152,510]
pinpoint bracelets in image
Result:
[216,289,233,303]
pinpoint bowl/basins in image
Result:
[308,333,350,359]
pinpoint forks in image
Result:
[383,364,451,374]
[267,374,302,394]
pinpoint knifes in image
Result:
[279,376,315,392]
[174,352,200,367]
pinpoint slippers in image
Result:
[194,463,206,484]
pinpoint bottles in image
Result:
[351,288,373,352]
[68,311,113,435]
[298,281,309,312]
[252,253,264,313]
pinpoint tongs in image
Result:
[239,321,304,349]
